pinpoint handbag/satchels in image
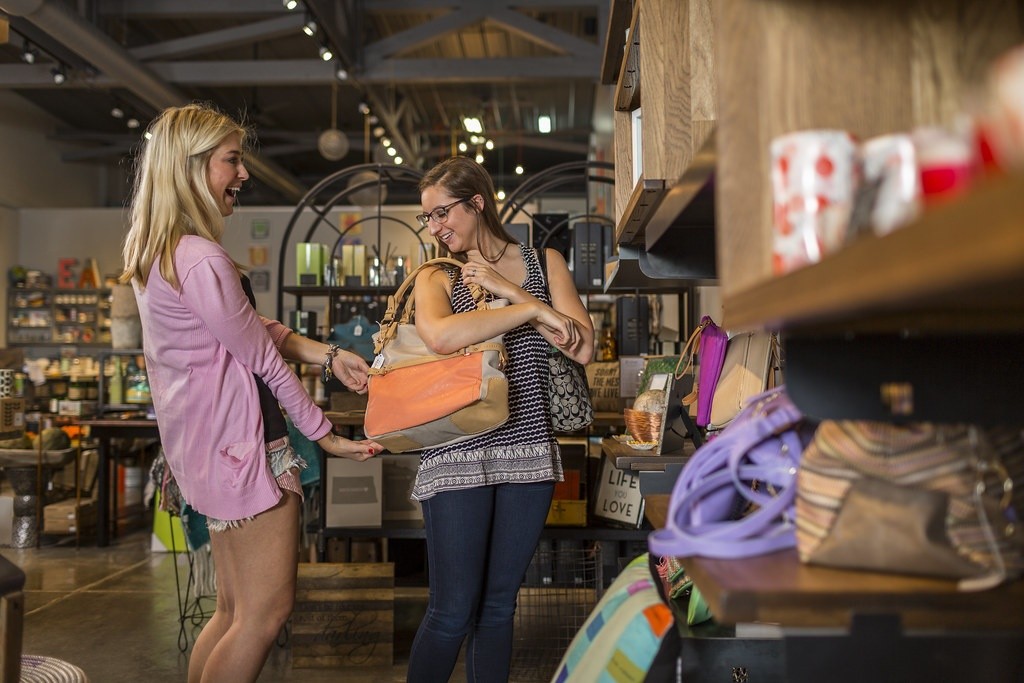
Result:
[710,330,784,428]
[795,382,1024,592]
[364,257,511,453]
[537,248,595,432]
[648,384,812,560]
[675,316,729,426]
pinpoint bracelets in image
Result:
[322,344,338,383]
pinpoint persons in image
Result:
[404,155,594,683]
[123,106,387,683]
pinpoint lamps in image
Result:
[346,114,387,209]
[281,0,298,10]
[20,40,40,65]
[302,21,317,37]
[318,81,351,161]
[48,65,68,85]
[319,46,332,61]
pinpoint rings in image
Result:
[473,269,476,276]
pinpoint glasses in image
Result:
[416,199,464,227]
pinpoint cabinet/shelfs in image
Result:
[643,170,1024,628]
[275,161,698,598]
[4,285,112,350]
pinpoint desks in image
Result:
[73,421,158,548]
